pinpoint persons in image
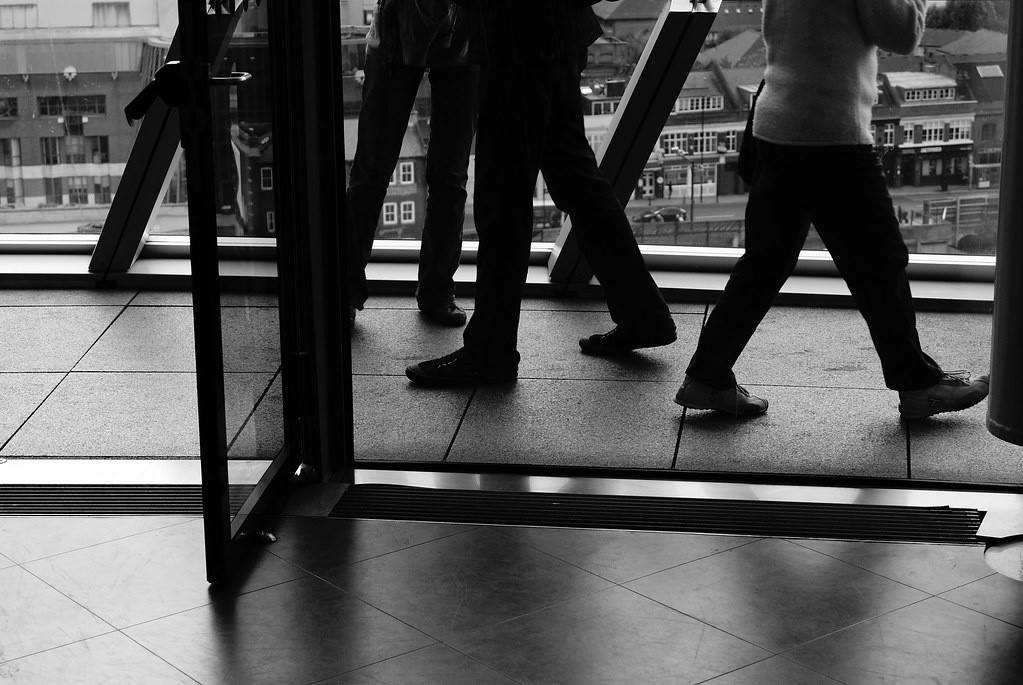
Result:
[675,0,990,420]
[339,0,677,383]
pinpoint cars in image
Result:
[78,222,103,234]
[633,204,687,223]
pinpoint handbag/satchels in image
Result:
[738,78,765,189]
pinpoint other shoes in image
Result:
[405,343,519,391]
[900,367,990,423]
[416,286,466,327]
[579,309,678,355]
[349,301,356,328]
[673,371,767,418]
